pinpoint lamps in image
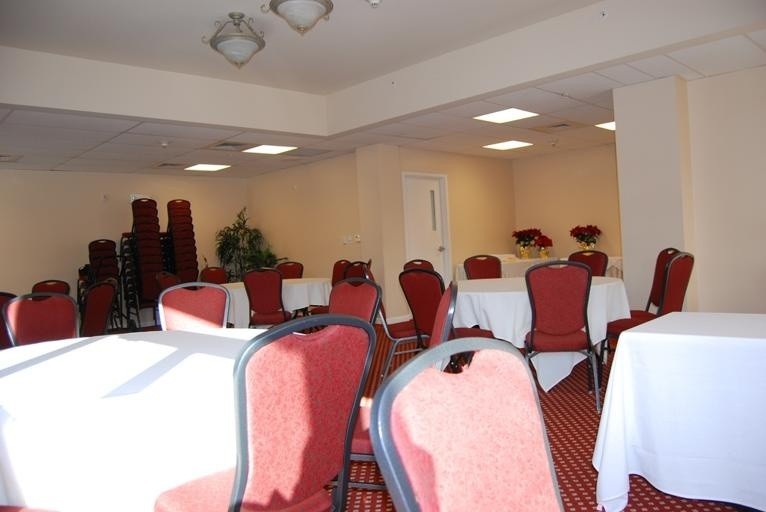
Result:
[201,13,266,68]
[260,0,334,34]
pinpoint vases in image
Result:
[538,247,552,260]
[575,242,597,251]
[514,246,533,259]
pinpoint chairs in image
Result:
[322,278,382,338]
[200,267,228,285]
[78,283,115,332]
[2,293,79,343]
[397,269,458,352]
[331,258,373,282]
[243,271,292,332]
[77,238,123,333]
[30,280,68,298]
[567,251,608,275]
[366,336,574,512]
[599,246,694,390]
[523,261,601,403]
[116,197,165,329]
[274,263,310,317]
[150,316,379,508]
[462,254,502,281]
[159,200,199,323]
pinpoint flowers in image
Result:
[568,223,600,240]
[515,227,554,247]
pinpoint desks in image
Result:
[202,277,334,335]
[0,326,315,512]
[450,275,626,391]
[591,311,766,512]
